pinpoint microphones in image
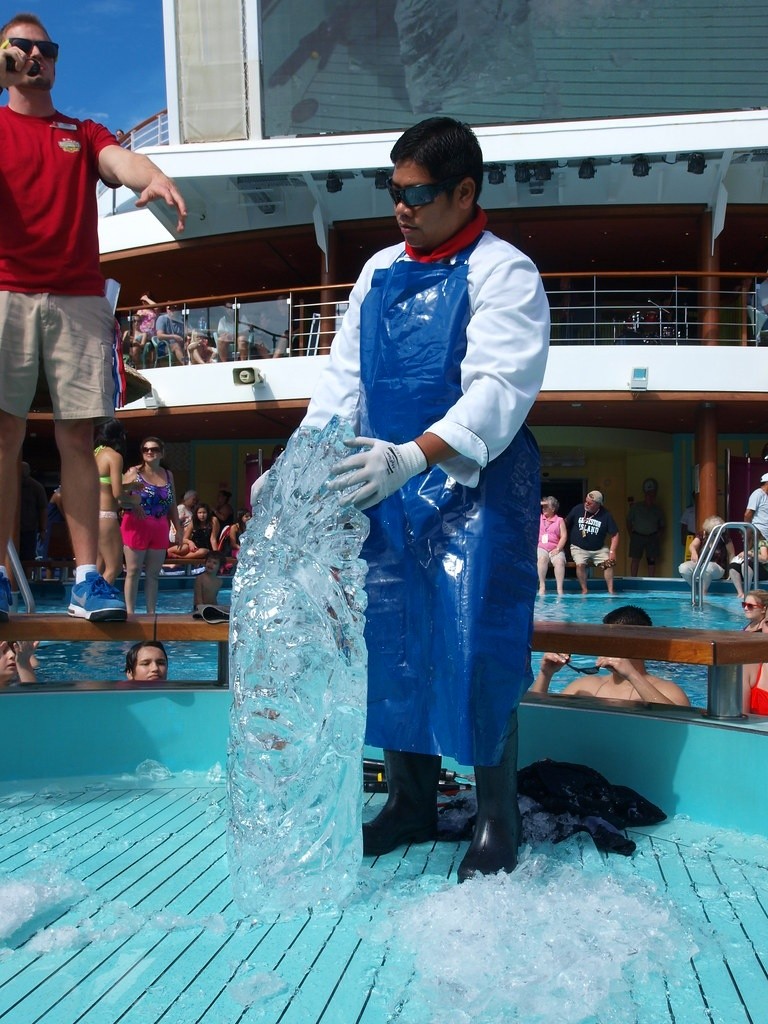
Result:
[5,56,41,77]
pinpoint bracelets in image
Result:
[556,547,561,552]
[610,550,616,554]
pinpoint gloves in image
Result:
[326,437,429,511]
[249,470,269,507]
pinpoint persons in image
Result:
[533,474,768,715]
[0,12,300,688]
[246,116,551,884]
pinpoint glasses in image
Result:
[742,602,759,611]
[0,37,60,60]
[225,306,235,310]
[385,174,450,208]
[565,654,600,674]
[142,447,161,454]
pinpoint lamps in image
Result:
[533,162,552,180]
[487,164,507,183]
[514,162,529,183]
[630,154,652,175]
[326,170,344,193]
[375,169,389,189]
[687,152,708,174]
[578,157,597,178]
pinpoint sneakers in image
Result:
[66,572,128,621]
[0,571,13,619]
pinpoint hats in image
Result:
[166,303,180,309]
[642,478,658,492]
[761,474,768,483]
[588,491,604,506]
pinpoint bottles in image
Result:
[200,317,206,331]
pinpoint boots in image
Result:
[458,708,520,882]
[361,748,441,857]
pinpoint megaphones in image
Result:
[232,367,262,385]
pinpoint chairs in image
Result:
[121,329,254,368]
